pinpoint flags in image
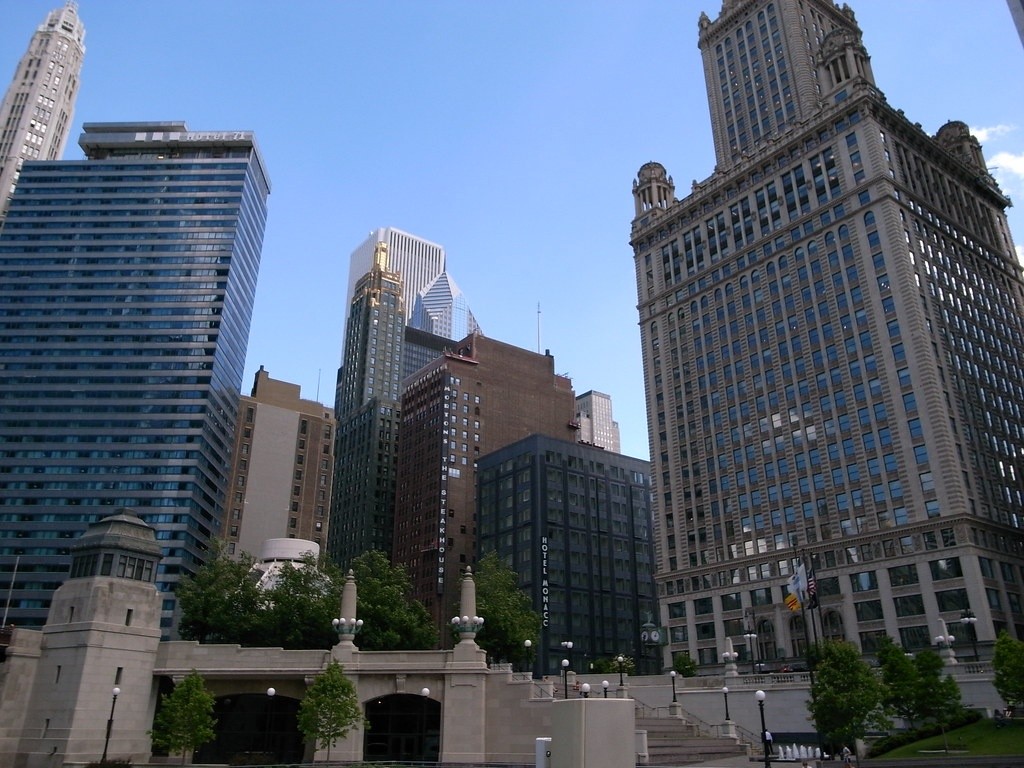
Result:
[786,566,817,613]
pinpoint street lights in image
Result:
[258,686,276,759]
[958,607,983,672]
[755,690,773,768]
[97,687,121,765]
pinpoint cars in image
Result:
[777,662,809,681]
